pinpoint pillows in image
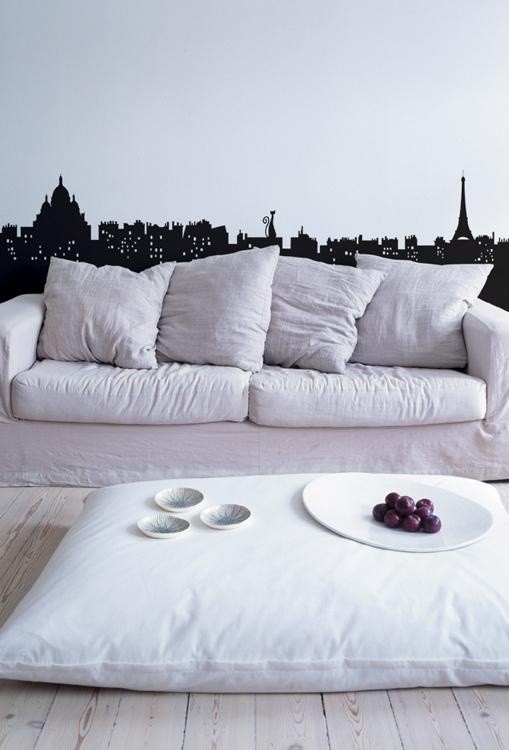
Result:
[157,245,282,369]
[37,258,177,368]
[0,474,509,693]
[266,254,390,370]
[349,251,495,368]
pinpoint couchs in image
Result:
[1,291,509,488]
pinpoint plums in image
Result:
[372,493,441,534]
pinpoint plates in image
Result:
[200,503,252,530]
[136,515,191,539]
[153,486,204,511]
[301,472,493,554]
